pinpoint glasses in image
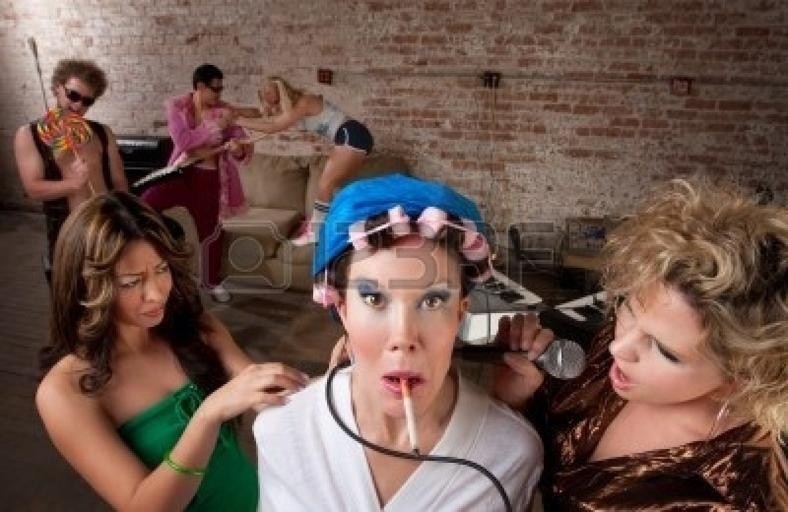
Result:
[62,86,95,107]
[209,86,222,92]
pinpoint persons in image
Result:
[219,76,373,246]
[251,174,544,512]
[488,176,788,512]
[139,63,255,302]
[13,59,129,288]
[35,191,310,512]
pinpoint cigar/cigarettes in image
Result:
[400,379,420,459]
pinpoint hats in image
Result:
[311,174,487,277]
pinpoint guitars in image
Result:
[134,133,273,188]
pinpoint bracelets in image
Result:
[164,450,210,476]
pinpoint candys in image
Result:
[36,106,99,196]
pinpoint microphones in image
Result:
[459,339,587,381]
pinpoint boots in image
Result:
[290,200,329,247]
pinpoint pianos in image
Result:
[539,287,618,355]
[456,269,545,349]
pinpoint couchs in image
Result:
[162,146,407,295]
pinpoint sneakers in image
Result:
[211,285,231,303]
